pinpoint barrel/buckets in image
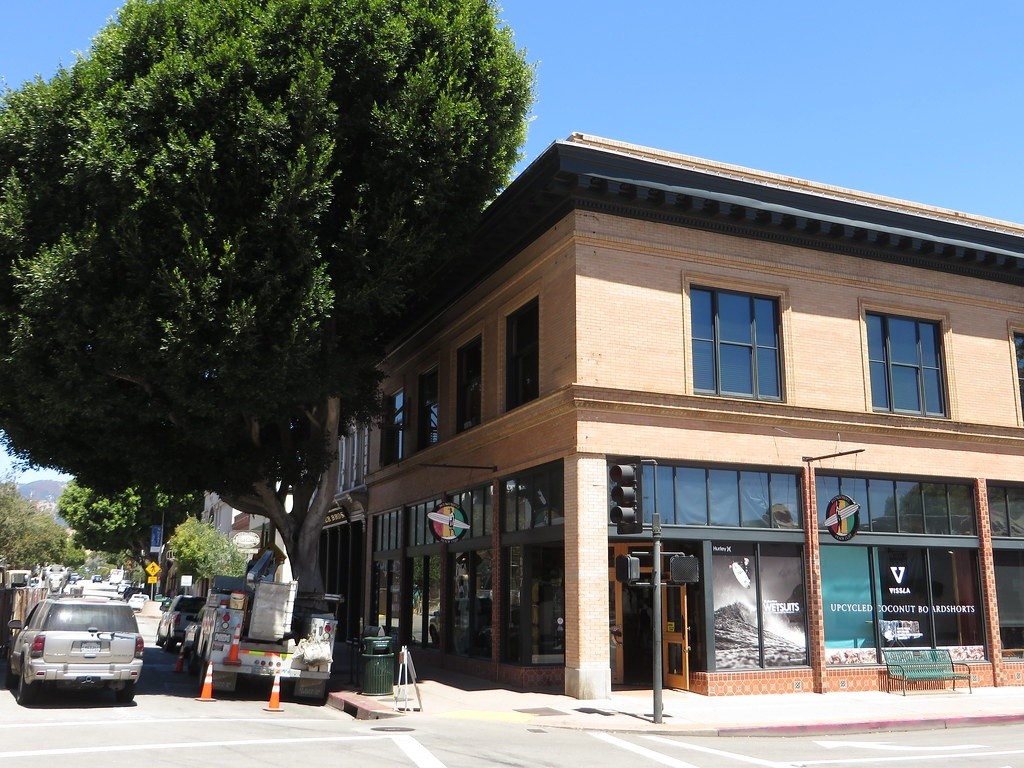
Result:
[229,592,245,610]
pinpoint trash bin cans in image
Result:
[361,635,394,696]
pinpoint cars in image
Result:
[106,569,147,612]
[7,564,82,589]
[92,575,103,583]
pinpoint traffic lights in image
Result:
[609,455,643,534]
[614,554,641,583]
[669,554,700,584]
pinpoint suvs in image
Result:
[429,596,494,646]
[153,595,207,651]
[4,593,145,707]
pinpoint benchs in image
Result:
[347,624,381,656]
[881,648,973,696]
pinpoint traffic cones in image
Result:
[221,624,243,667]
[170,646,187,674]
[195,660,218,702]
[261,670,285,713]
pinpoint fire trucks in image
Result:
[182,544,344,705]
